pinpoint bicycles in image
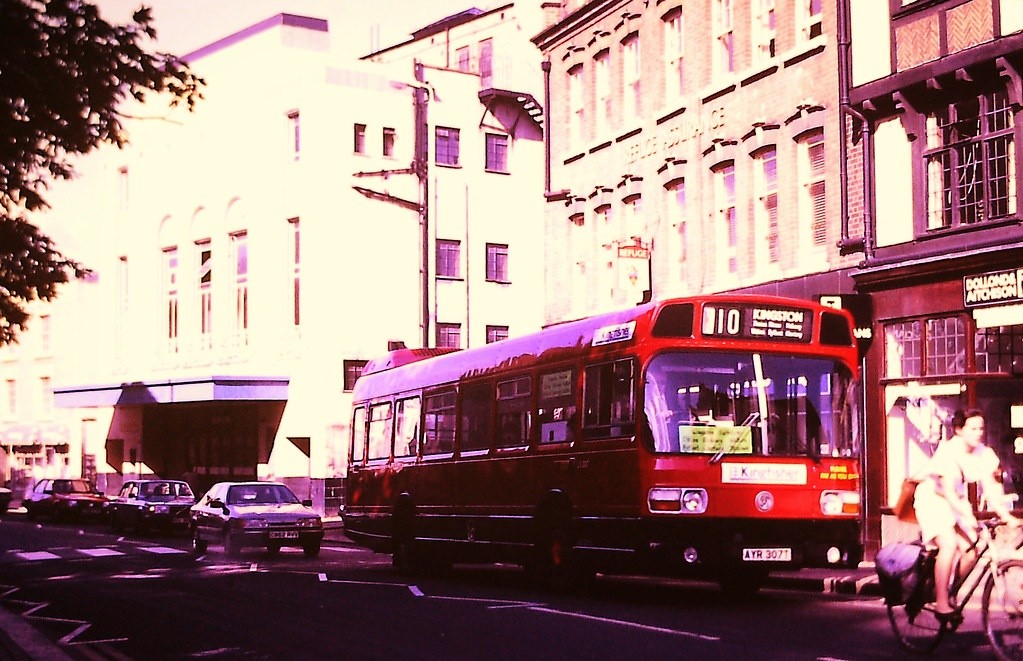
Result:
[883,510,1023,661]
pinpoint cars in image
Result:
[101,479,198,538]
[189,481,324,558]
[21,478,110,526]
[0,487,13,516]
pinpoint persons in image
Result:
[914,408,1023,622]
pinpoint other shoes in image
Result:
[934,608,964,621]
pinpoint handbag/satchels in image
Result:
[891,478,920,523]
[876,541,923,607]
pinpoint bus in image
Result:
[335,296,865,609]
